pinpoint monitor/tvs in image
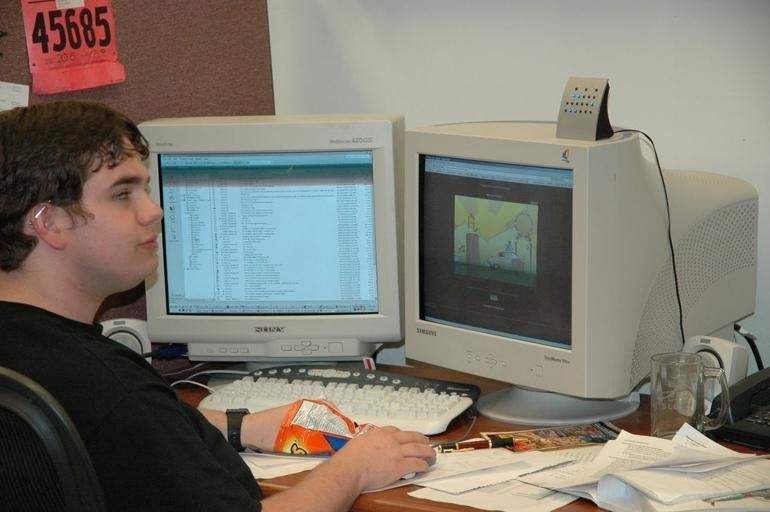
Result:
[137,113,406,395]
[405,120,759,427]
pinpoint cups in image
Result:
[648,351,730,442]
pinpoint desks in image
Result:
[152,356,769,512]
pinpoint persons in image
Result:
[0,102,437,512]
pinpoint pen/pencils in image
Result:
[438,436,528,454]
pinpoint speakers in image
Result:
[99,318,151,365]
[676,335,750,418]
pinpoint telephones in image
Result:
[707,367,770,447]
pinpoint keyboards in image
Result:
[197,365,481,436]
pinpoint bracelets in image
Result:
[223,405,254,453]
